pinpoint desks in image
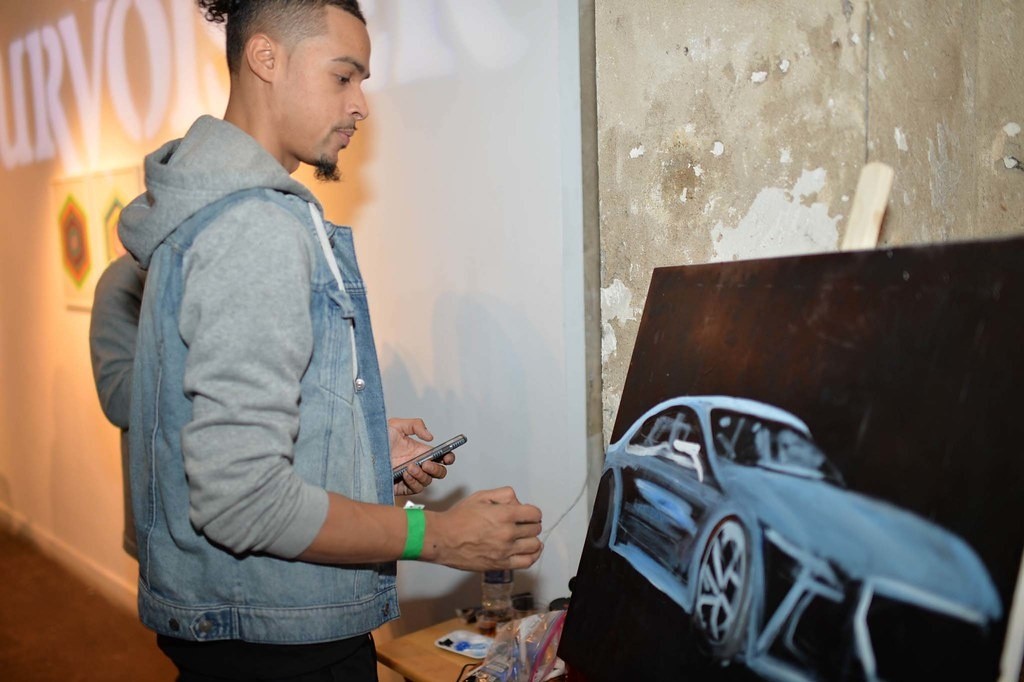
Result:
[375,609,534,682]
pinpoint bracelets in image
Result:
[400,500,427,561]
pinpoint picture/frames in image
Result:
[47,163,145,313]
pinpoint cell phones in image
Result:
[394,434,467,483]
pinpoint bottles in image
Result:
[482,569,514,621]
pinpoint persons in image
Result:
[117,1,549,682]
[87,252,150,560]
